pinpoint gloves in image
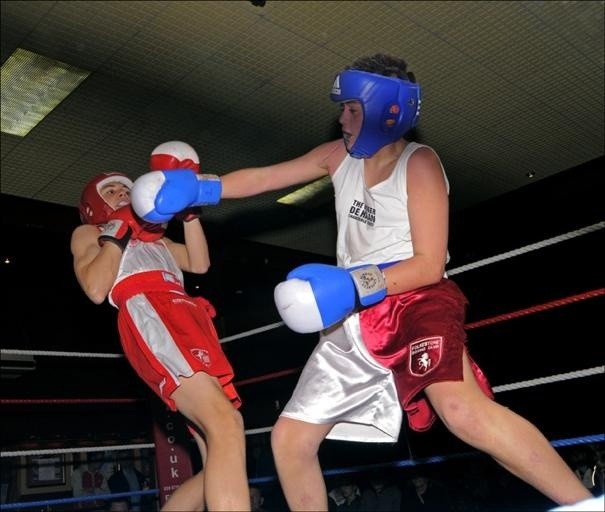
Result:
[99,140,221,252]
[274,264,388,335]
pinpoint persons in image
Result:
[572,442,605,497]
[129,51,595,512]
[70,140,252,512]
[72,447,141,512]
[328,468,462,512]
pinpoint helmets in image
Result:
[79,171,133,224]
[331,67,421,159]
